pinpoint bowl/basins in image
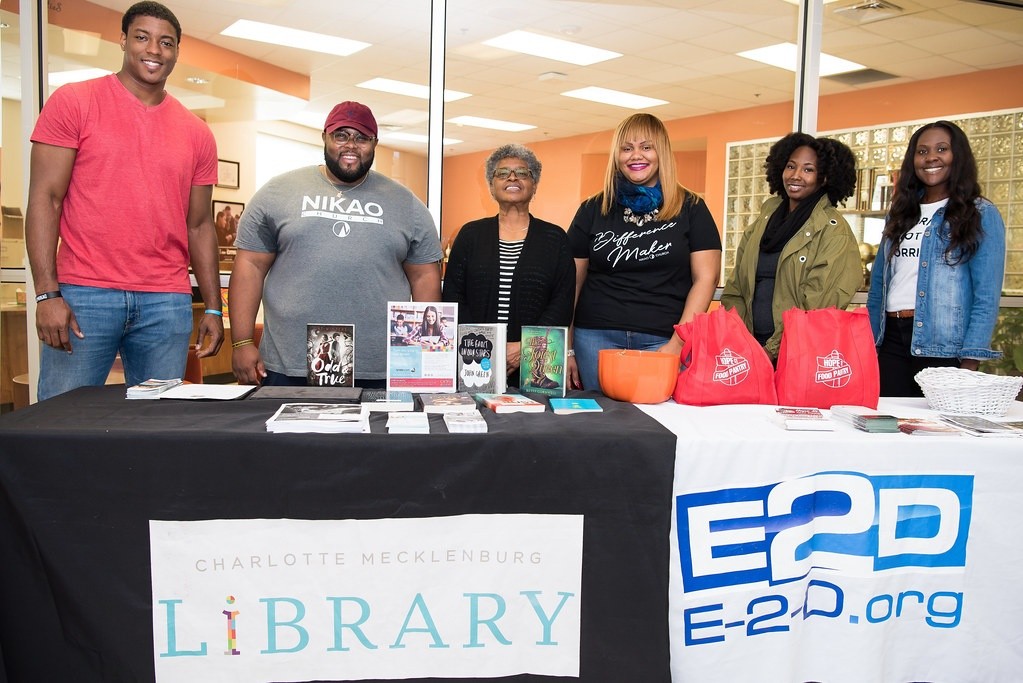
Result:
[598,349,680,404]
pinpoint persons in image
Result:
[566,114,723,392]
[865,121,1006,397]
[720,133,863,372]
[443,143,576,388]
[215,206,243,247]
[391,306,450,345]
[25,0,225,402]
[313,333,343,373]
[228,101,444,389]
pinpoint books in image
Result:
[387,301,458,394]
[520,326,568,398]
[458,323,507,394]
[778,405,1023,439]
[361,390,604,434]
[307,323,355,387]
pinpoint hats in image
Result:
[324,101,378,138]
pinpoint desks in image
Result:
[0,387,1023,683]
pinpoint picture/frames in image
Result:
[212,201,244,247]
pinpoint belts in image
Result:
[886,310,915,318]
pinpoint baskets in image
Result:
[914,367,1022,419]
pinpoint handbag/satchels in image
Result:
[775,307,880,412]
[674,306,779,406]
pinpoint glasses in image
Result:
[332,131,372,147]
[493,168,534,180]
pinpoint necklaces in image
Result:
[499,222,528,233]
[325,166,368,198]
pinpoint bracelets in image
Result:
[232,339,254,348]
[36,291,63,304]
[205,309,223,317]
[568,349,575,356]
